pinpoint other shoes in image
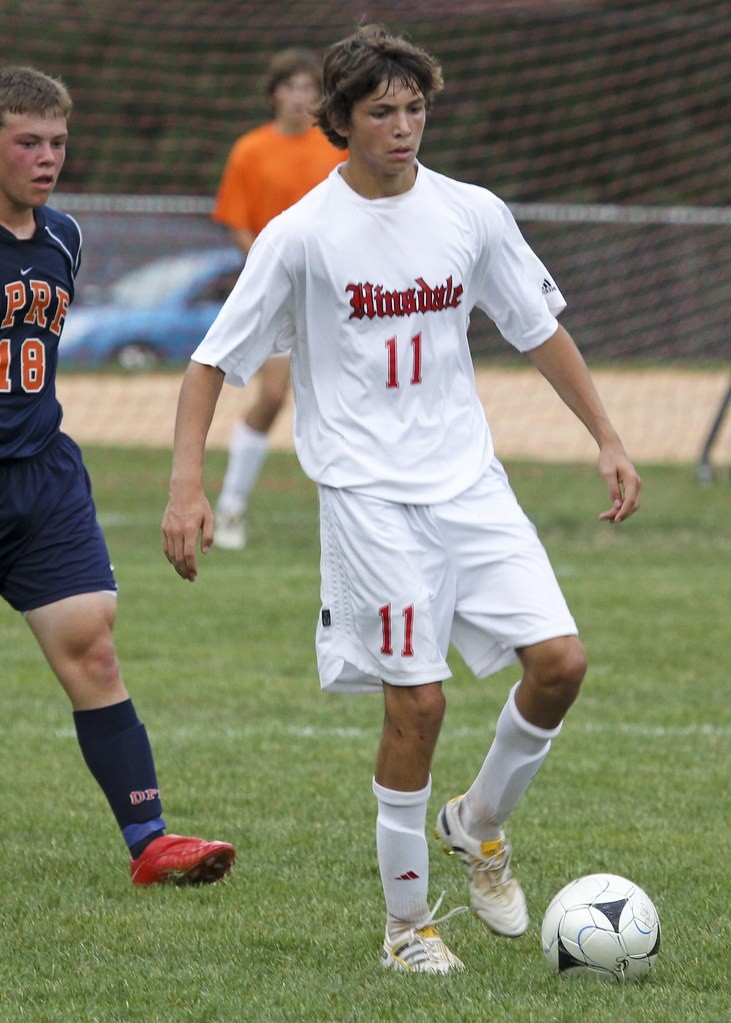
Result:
[209,508,248,551]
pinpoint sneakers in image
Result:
[380,891,466,977]
[433,794,530,937]
[128,834,236,889]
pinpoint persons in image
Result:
[0,64,236,892]
[162,23,641,974]
[212,44,348,552]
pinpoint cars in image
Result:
[54,247,295,369]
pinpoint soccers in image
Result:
[540,873,662,982]
